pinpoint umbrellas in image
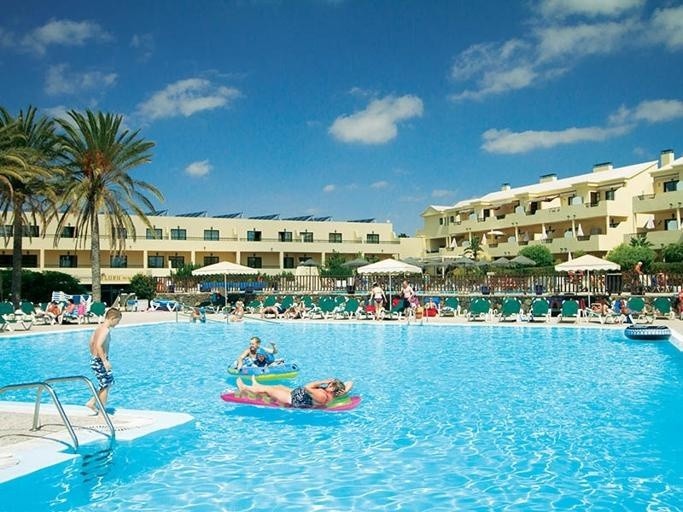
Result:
[298,252,537,310]
[567,251,576,274]
[554,254,621,309]
[577,223,584,236]
[481,234,486,244]
[450,237,457,248]
[486,231,504,236]
[191,260,259,306]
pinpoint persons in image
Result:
[235,337,278,372]
[357,279,437,321]
[250,349,283,370]
[235,374,352,408]
[86,309,123,416]
[42,294,94,326]
[593,261,683,324]
[188,280,307,322]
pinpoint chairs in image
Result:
[0,298,107,332]
[466,295,673,324]
[195,292,460,319]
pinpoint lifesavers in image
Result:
[220,386,360,411]
[625,325,671,339]
[227,358,299,379]
